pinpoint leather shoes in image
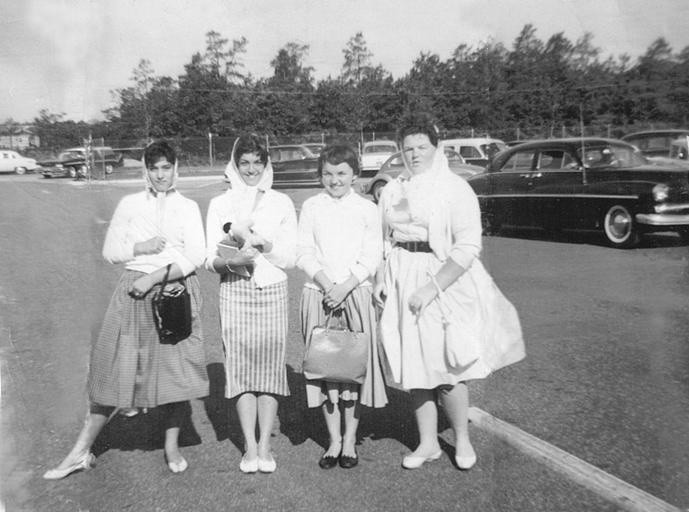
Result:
[258,458,277,473]
[454,451,478,470]
[339,447,359,468]
[239,456,259,473]
[318,448,343,469]
[42,454,92,480]
[401,449,442,469]
[167,456,188,473]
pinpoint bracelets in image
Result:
[225,257,236,273]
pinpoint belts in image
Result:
[395,240,431,253]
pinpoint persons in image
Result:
[206,136,300,474]
[42,140,206,478]
[373,125,527,469]
[297,145,382,468]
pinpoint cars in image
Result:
[268,143,326,190]
[464,138,689,248]
[356,141,399,175]
[617,130,689,159]
[0,151,39,174]
[437,139,512,162]
[38,146,117,178]
[362,149,487,203]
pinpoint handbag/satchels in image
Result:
[300,305,370,386]
[426,271,482,370]
[150,264,193,346]
